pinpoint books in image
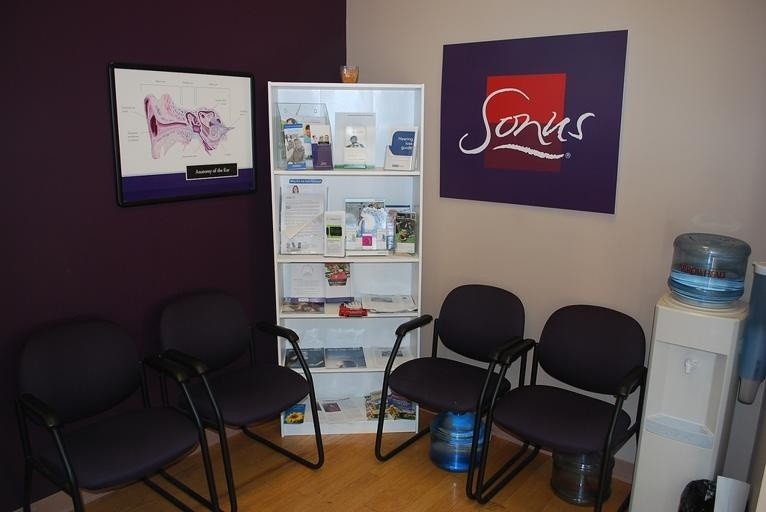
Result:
[285,349,413,370]
[282,262,417,314]
[279,103,417,170]
[280,185,416,256]
[285,392,415,423]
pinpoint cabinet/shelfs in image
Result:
[267,81,426,438]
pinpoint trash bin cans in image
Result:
[677,479,716,512]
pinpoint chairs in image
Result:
[155,289,326,511]
[475,303,649,510]
[373,282,529,501]
[12,315,220,511]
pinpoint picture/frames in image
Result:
[107,62,257,207]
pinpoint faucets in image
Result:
[683,358,697,372]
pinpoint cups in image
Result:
[341,65,359,82]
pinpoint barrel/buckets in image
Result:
[549,456,613,504]
[667,232,751,308]
[429,410,488,473]
